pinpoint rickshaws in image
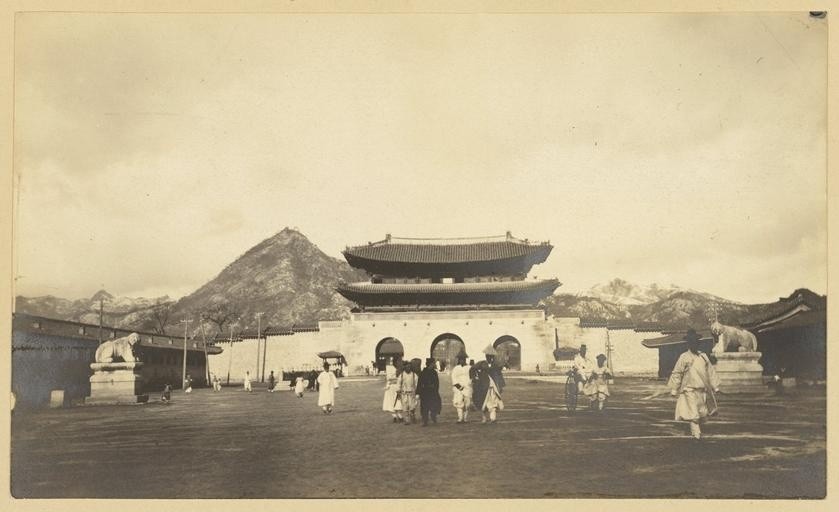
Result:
[564,369,613,411]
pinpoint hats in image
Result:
[482,343,499,356]
[455,347,469,358]
[322,362,332,367]
[682,328,702,340]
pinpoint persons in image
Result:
[244,370,252,392]
[582,353,617,411]
[185,373,193,394]
[315,362,340,416]
[668,328,720,441]
[294,371,305,399]
[162,379,172,404]
[384,353,507,427]
[217,377,222,391]
[369,360,374,372]
[574,341,594,382]
[213,374,218,392]
[266,369,275,393]
[372,360,377,377]
